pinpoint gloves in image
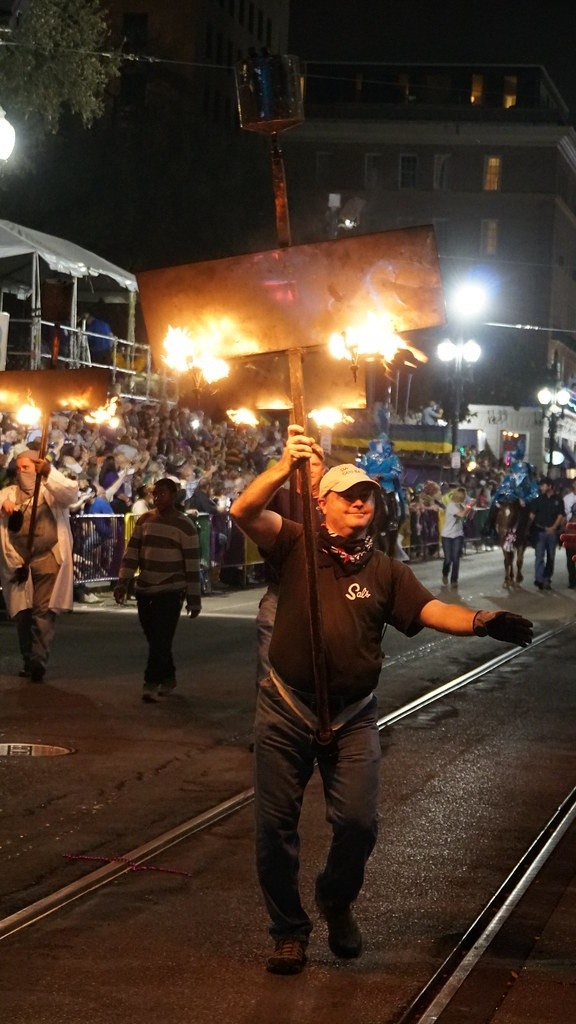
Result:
[473,610,534,647]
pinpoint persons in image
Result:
[60,312,117,375]
[0,451,80,681]
[0,347,286,603]
[232,424,535,972]
[317,399,576,590]
[114,479,202,702]
[248,440,327,758]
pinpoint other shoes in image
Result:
[535,580,544,591]
[143,684,158,701]
[316,877,362,956]
[266,935,308,973]
[442,575,448,585]
[451,582,457,588]
[30,659,46,682]
[544,581,551,590]
[157,679,176,696]
[19,665,32,677]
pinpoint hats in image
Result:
[536,477,555,487]
[318,463,380,499]
[311,443,326,461]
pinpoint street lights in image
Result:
[330,310,404,432]
[436,280,483,456]
[538,361,571,482]
[164,316,256,413]
